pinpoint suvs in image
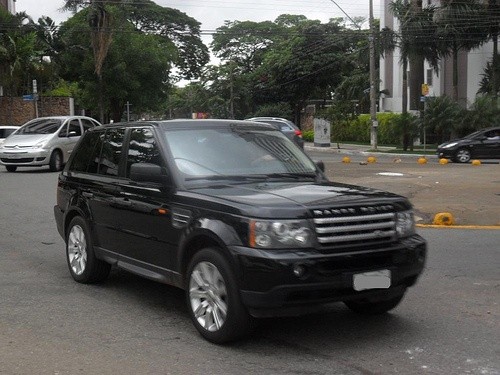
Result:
[245,117,303,151]
[54,118,427,344]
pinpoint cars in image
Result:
[437,126,500,163]
[0,115,102,173]
